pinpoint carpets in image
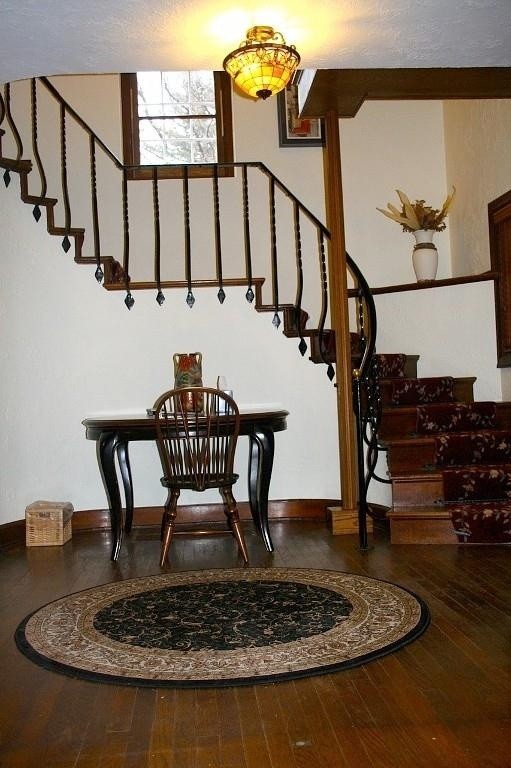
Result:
[14,565,431,689]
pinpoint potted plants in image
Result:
[375,186,455,282]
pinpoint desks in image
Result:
[80,409,287,562]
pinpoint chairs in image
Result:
[149,386,250,566]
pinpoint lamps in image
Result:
[221,23,302,98]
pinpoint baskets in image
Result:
[26,501,74,547]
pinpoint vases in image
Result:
[173,352,204,413]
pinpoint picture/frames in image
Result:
[277,86,325,147]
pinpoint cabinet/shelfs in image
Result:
[487,190,511,368]
